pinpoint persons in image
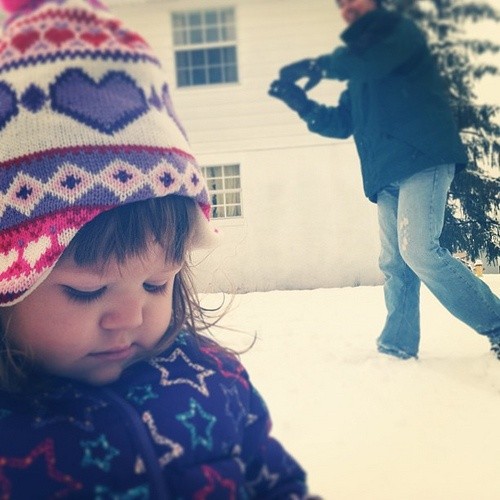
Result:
[0,0,307,499]
[269,0,500,359]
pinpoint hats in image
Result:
[0,0,225,306]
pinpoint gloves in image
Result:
[279,58,321,89]
[268,80,319,118]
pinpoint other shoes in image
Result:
[492,336,500,360]
